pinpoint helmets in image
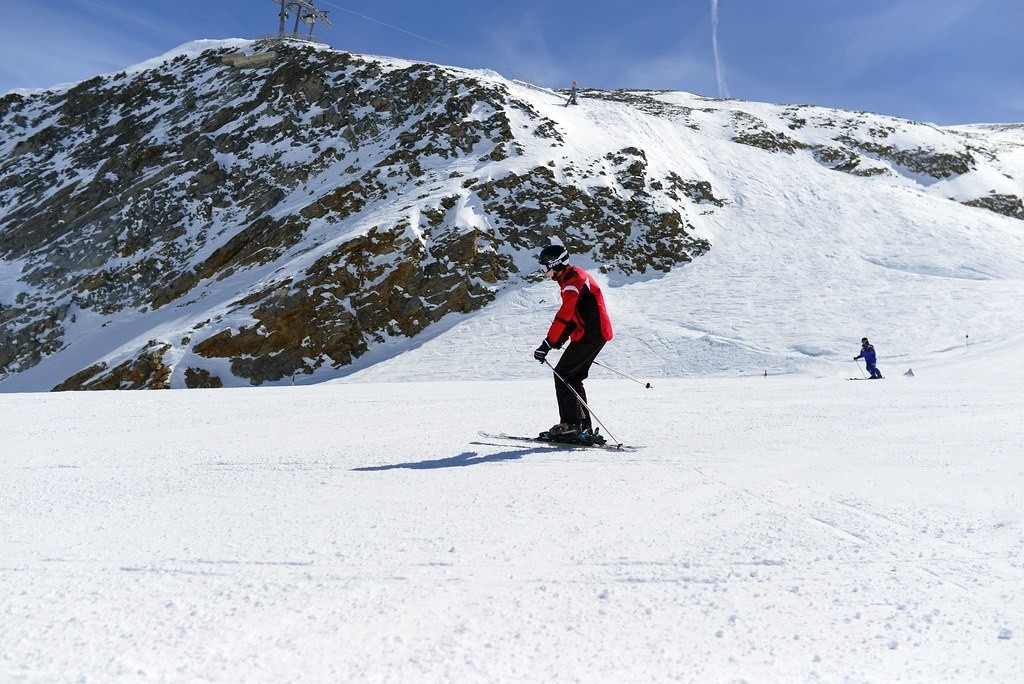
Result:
[862,338,868,344]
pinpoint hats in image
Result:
[538,244,571,272]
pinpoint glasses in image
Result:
[539,264,548,272]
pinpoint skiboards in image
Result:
[845,376,886,381]
[474,428,648,455]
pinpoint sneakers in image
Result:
[549,419,592,436]
[869,375,882,379]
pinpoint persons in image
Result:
[570,82,579,105]
[533,246,612,433]
[853,338,882,378]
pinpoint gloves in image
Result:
[854,357,856,360]
[555,341,562,350]
[534,337,553,364]
